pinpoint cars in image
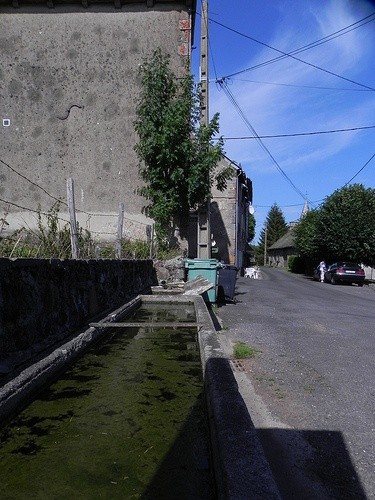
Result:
[311,265,331,278]
[325,262,365,287]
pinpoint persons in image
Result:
[319,258,327,283]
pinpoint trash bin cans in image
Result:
[218,264,240,303]
